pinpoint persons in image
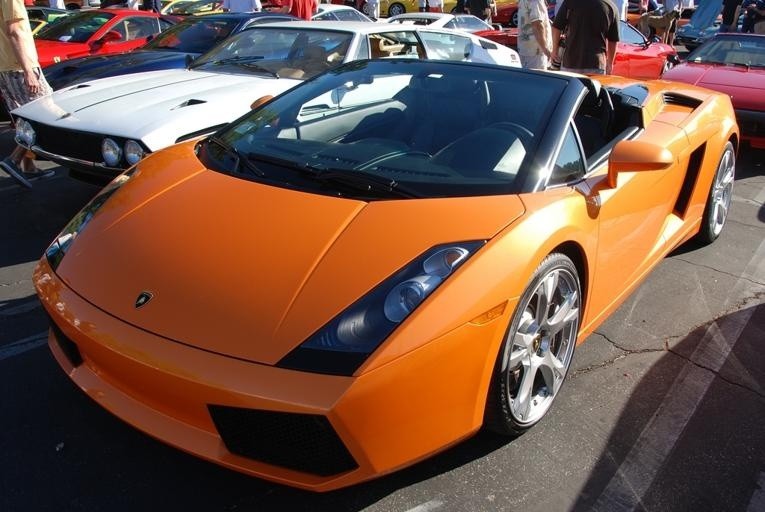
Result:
[517,0,553,71]
[460,1,497,26]
[720,1,744,35]
[742,1,765,34]
[662,1,682,50]
[270,0,319,22]
[224,0,262,15]
[550,1,620,75]
[143,0,162,14]
[616,1,629,23]
[0,0,56,192]
[638,0,649,16]
[638,0,660,42]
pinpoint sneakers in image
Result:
[0,157,33,189]
[25,168,55,182]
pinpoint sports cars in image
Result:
[22,0,724,68]
[34,57,740,492]
[659,32,765,149]
[1,20,524,187]
[472,17,681,81]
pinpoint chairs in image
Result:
[409,71,490,128]
[541,75,617,144]
